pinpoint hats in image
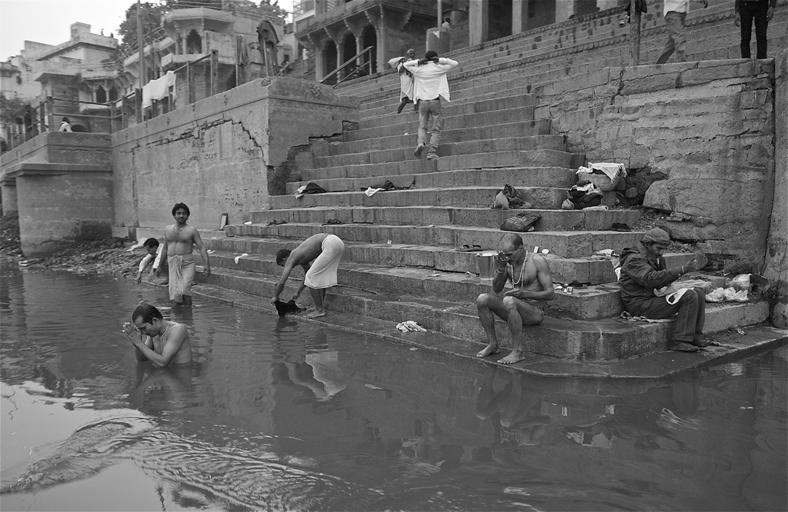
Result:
[642,227,670,244]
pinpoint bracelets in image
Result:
[680,264,686,274]
[494,268,505,278]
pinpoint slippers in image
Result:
[460,245,472,252]
[472,244,483,251]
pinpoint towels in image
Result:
[140,72,178,110]
[303,233,345,289]
[575,160,628,184]
[167,253,196,301]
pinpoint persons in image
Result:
[442,17,450,27]
[615,385,699,429]
[735,0,777,59]
[620,226,708,352]
[168,307,213,377]
[122,303,193,368]
[59,118,72,133]
[302,48,310,74]
[477,234,554,364]
[475,365,550,466]
[136,238,166,285]
[274,318,347,415]
[396,48,415,114]
[157,203,210,308]
[270,232,345,318]
[403,51,458,160]
[129,369,191,431]
[656,0,708,63]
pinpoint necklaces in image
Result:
[511,249,529,290]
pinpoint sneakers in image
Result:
[414,143,424,156]
[612,223,619,230]
[705,288,726,303]
[694,340,707,347]
[427,152,440,159]
[671,341,699,352]
[617,224,631,232]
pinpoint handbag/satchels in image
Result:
[500,212,542,232]
